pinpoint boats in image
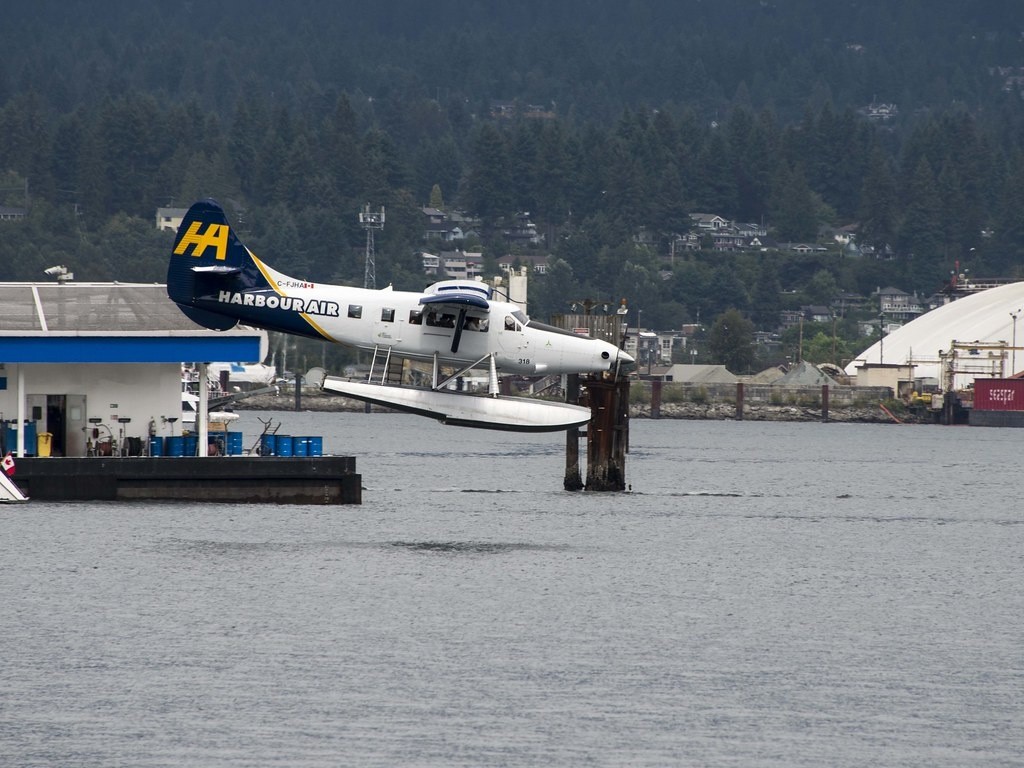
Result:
[181,392,240,423]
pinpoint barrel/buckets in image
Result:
[148,437,163,457]
[184,431,242,456]
[165,436,183,456]
[261,434,323,456]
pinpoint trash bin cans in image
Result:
[37,433,53,457]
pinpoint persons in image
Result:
[411,312,485,331]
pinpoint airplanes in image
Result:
[165,198,635,434]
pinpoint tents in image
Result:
[206,362,276,388]
